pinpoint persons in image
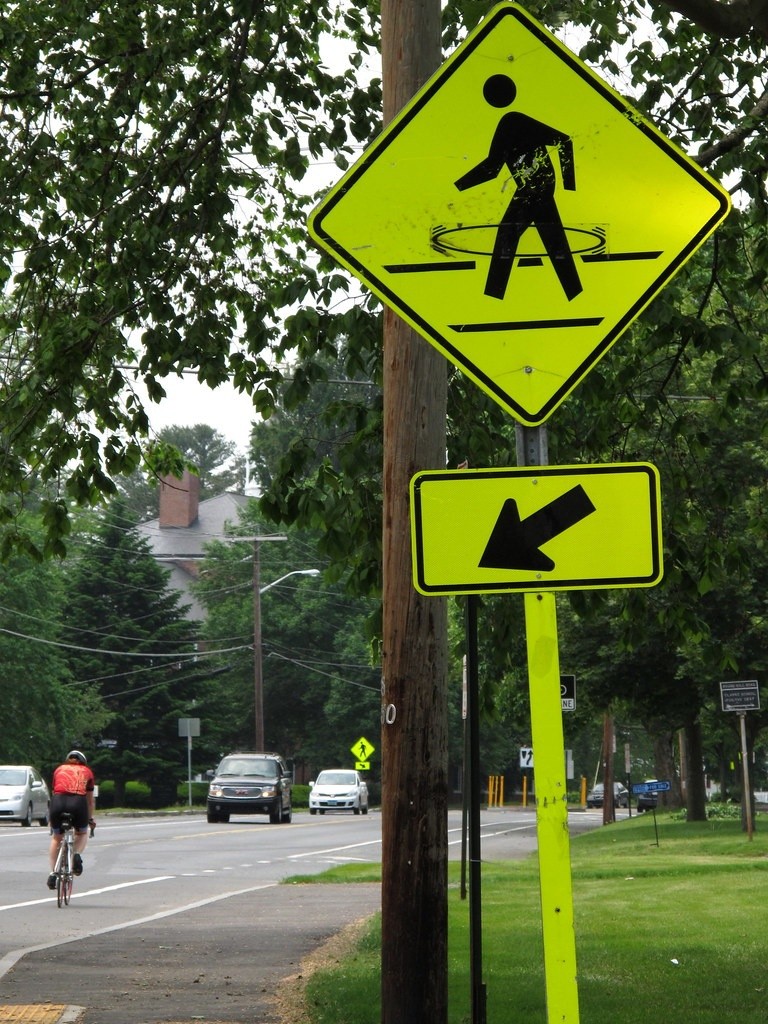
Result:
[47,750,96,890]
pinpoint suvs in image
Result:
[206,752,293,825]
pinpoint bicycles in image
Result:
[46,798,96,909]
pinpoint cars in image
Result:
[586,781,629,809]
[637,779,661,813]
[308,769,368,816]
[0,765,51,828]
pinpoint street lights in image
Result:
[255,569,321,754]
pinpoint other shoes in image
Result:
[71,852,83,876]
[47,876,56,887]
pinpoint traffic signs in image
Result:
[721,679,760,710]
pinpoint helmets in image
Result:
[66,750,87,765]
